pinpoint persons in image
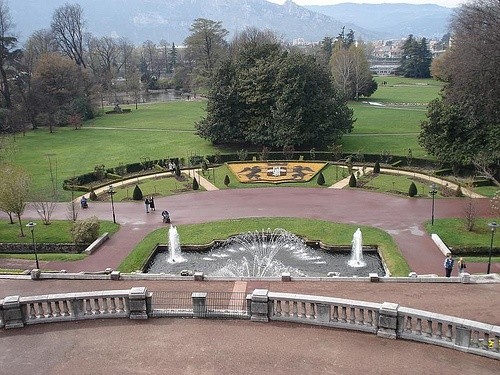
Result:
[457,257,467,274]
[444,256,454,277]
[145,195,155,213]
[81,196,88,208]
[162,210,170,223]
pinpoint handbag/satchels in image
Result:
[464,264,466,268]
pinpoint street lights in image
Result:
[25,222,40,268]
[106,185,116,224]
[429,183,437,225]
[487,222,499,274]
